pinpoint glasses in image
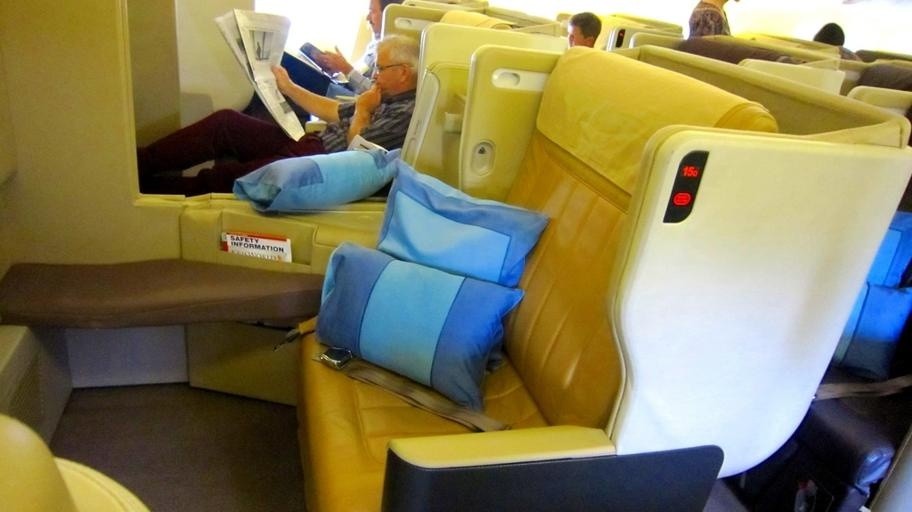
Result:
[375,63,403,75]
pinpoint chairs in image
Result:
[555,13,846,95]
[305,2,568,135]
[273,45,912,510]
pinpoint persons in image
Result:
[136,32,420,199]
[568,11,603,49]
[686,0,735,38]
[236,0,407,133]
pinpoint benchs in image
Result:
[1,259,327,331]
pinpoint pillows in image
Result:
[233,147,401,216]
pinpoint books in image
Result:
[299,40,343,79]
